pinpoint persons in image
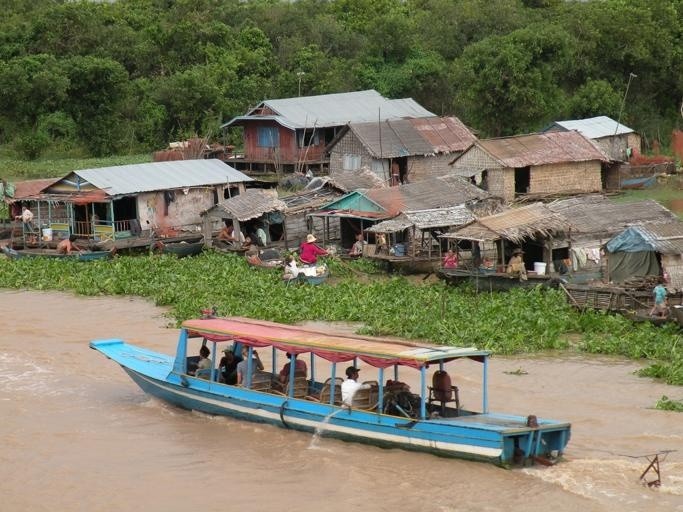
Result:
[648,278,668,318]
[444,249,458,269]
[390,160,400,186]
[299,234,328,266]
[217,220,267,248]
[340,366,377,410]
[506,248,526,273]
[56,235,81,255]
[470,175,476,185]
[217,345,243,385]
[188,346,212,376]
[236,345,264,387]
[354,234,368,256]
[15,203,37,245]
[284,255,325,277]
[279,352,308,389]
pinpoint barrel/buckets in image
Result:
[42,228,53,242]
[533,262,547,275]
[432,369,452,401]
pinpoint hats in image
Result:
[346,366,361,375]
[222,345,234,353]
[512,248,525,255]
[306,234,317,244]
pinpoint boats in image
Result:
[624,306,671,328]
[274,255,331,286]
[245,245,287,273]
[89,307,576,470]
[1,246,120,262]
[142,236,205,259]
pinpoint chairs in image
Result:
[195,367,402,414]
